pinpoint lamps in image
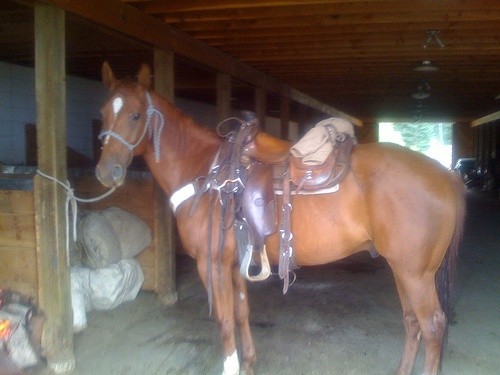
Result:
[402,30,449,109]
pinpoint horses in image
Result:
[93,61,468,375]
